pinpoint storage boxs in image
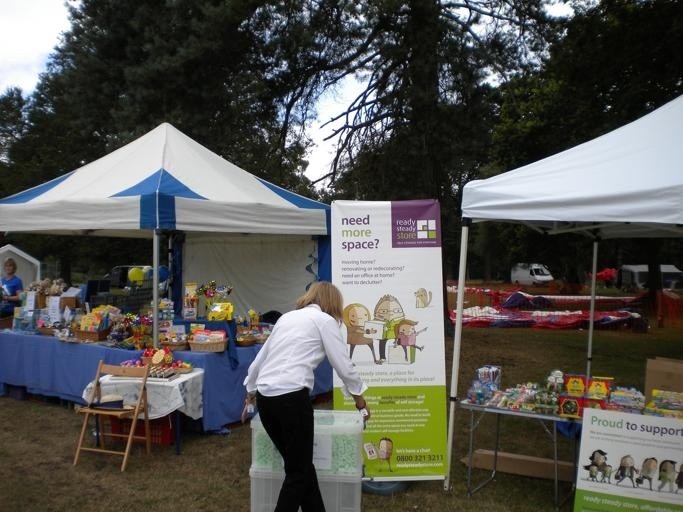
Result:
[250,411,364,478]
[250,476,363,512]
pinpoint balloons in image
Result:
[158,266,169,283]
[143,265,152,273]
[146,269,153,279]
[129,268,144,286]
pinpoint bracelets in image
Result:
[355,401,366,410]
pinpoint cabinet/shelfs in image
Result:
[462,393,584,501]
[0,329,333,437]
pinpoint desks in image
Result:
[88,368,204,456]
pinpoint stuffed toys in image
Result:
[26,277,67,296]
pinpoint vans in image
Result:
[624,265,683,297]
[511,263,554,288]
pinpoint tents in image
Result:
[445,96,683,492]
[0,122,331,434]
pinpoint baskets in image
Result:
[25,292,46,309]
[158,340,188,350]
[60,296,77,309]
[38,325,58,335]
[236,334,257,347]
[76,326,109,340]
[188,337,229,352]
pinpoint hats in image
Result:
[89,395,135,411]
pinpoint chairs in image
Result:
[71,359,152,473]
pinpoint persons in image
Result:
[241,282,366,512]
[0,259,23,320]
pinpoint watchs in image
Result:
[245,396,256,401]
